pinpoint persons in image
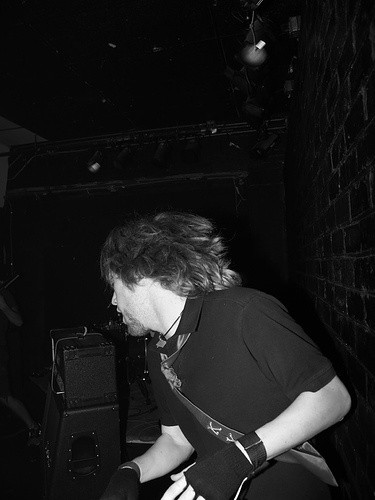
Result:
[0,280,42,447]
[98,212,351,500]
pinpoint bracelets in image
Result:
[116,461,140,479]
[237,430,267,467]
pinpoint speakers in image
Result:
[36,328,122,500]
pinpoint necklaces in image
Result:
[163,316,180,336]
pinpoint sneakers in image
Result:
[27,421,44,448]
